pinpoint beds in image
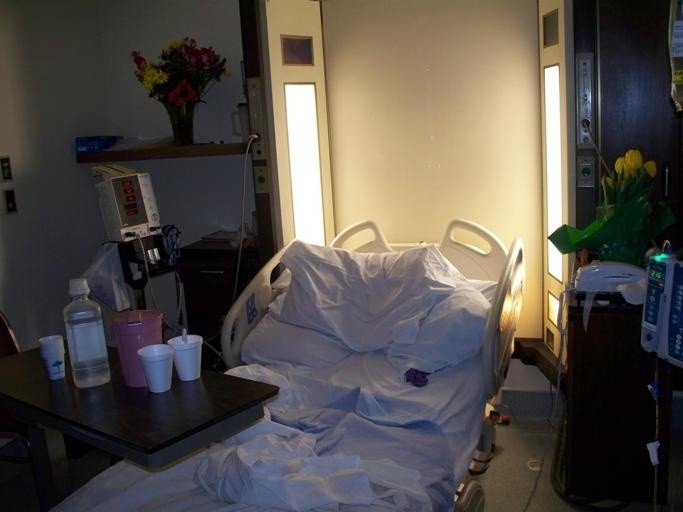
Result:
[48,216,528,512]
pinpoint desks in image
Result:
[1,343,281,512]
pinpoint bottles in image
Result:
[62,277,111,390]
[36,334,66,382]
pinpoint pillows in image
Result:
[267,237,497,379]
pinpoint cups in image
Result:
[167,334,203,382]
[228,101,250,144]
[107,308,166,391]
[136,344,175,394]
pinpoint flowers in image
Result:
[128,34,231,114]
[546,150,674,265]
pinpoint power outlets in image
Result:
[576,51,597,190]
[244,76,270,195]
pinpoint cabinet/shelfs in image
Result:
[174,233,261,346]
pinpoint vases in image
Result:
[160,99,198,145]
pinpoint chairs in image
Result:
[0,310,56,512]
